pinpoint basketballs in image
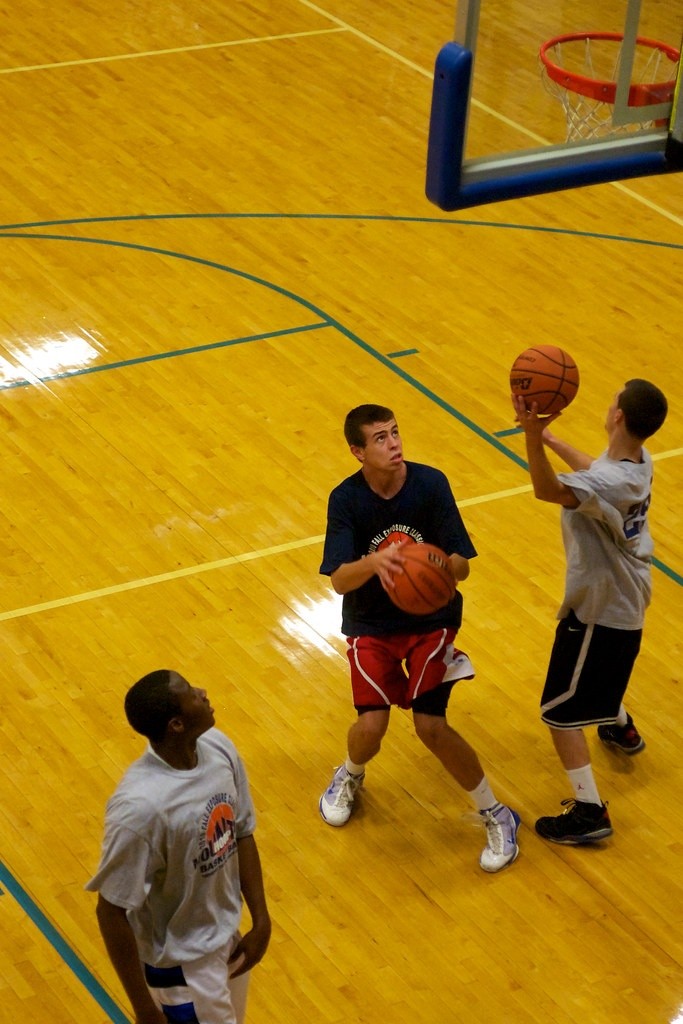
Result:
[386,541,455,616]
[508,345,578,412]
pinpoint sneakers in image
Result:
[597,712,646,757]
[534,800,614,845]
[319,762,366,826]
[479,801,523,874]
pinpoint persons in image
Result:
[510,379,670,846]
[85,668,274,1024]
[315,401,523,874]
